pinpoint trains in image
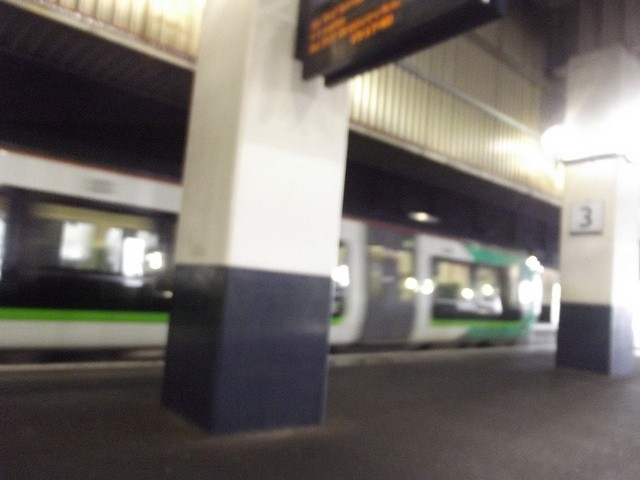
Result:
[0,148,544,349]
